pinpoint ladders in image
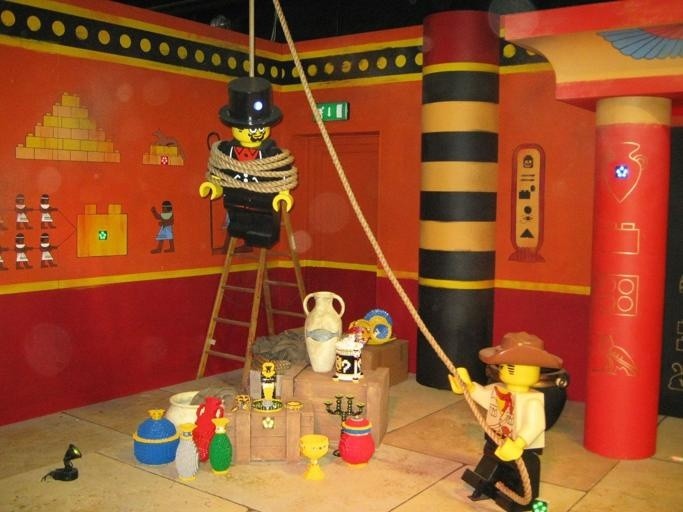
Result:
[196,200,309,391]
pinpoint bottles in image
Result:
[163,389,225,431]
[190,395,224,463]
[207,417,235,475]
[299,290,348,373]
[174,422,200,482]
[337,414,376,470]
[131,406,180,466]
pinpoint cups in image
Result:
[331,340,364,383]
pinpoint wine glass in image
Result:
[298,433,328,480]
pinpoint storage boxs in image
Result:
[232,339,410,466]
